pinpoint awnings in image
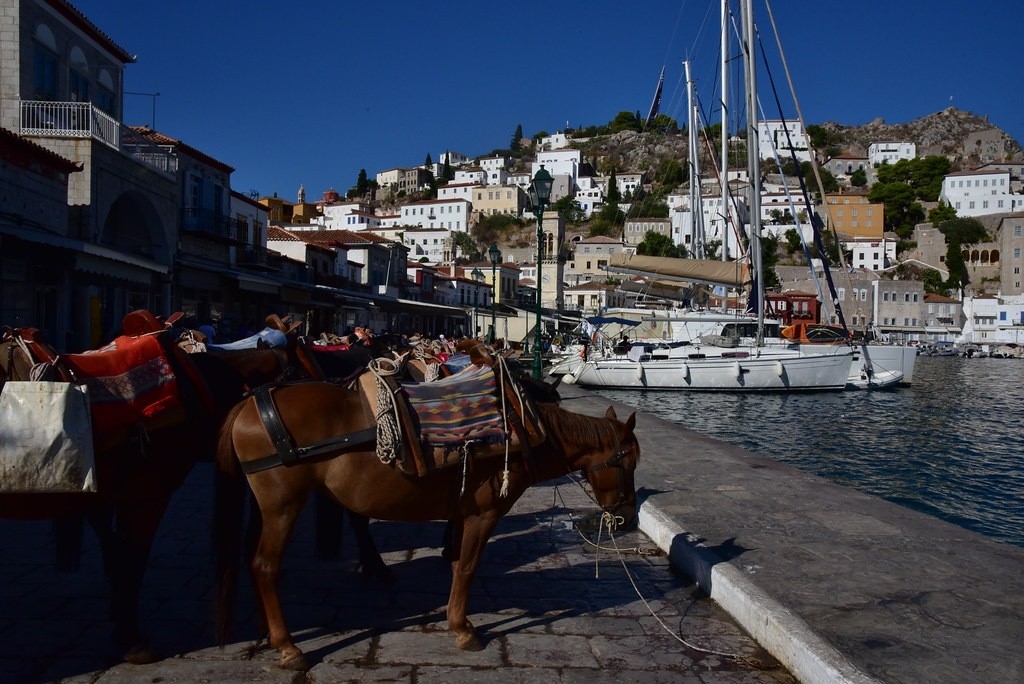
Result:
[0,219,169,287]
[177,259,465,313]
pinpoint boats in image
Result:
[905,337,1024,358]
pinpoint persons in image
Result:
[197,318,215,345]
[618,335,631,347]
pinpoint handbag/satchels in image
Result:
[0,362,104,492]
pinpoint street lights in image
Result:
[487,242,501,347]
[531,164,554,388]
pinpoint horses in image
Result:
[3,329,643,672]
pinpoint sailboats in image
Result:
[550,1,902,391]
[552,36,919,386]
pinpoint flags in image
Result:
[642,63,666,129]
